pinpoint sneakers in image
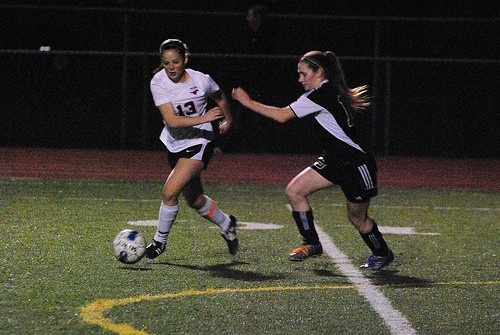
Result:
[145,239,166,258]
[288,243,323,262]
[360,249,394,274]
[221,215,238,255]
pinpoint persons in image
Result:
[230,48,396,272]
[145,37,241,258]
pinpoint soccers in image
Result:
[112,229,147,264]
